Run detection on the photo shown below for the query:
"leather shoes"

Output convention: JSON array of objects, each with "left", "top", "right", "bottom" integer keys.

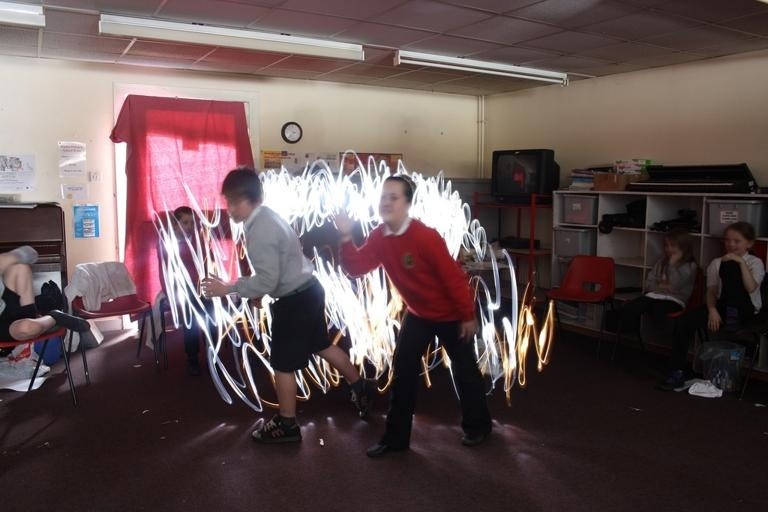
[
  {"left": 461, "top": 434, "right": 484, "bottom": 447},
  {"left": 365, "top": 441, "right": 389, "bottom": 459}
]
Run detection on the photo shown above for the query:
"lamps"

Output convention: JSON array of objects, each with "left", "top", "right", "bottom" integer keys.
[
  {"left": 97, "top": 14, "right": 365, "bottom": 65},
  {"left": 0, "top": 1, "right": 46, "bottom": 30},
  {"left": 393, "top": 50, "right": 570, "bottom": 87}
]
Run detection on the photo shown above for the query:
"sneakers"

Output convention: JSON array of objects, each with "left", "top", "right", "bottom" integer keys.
[
  {"left": 48, "top": 309, "right": 90, "bottom": 334},
  {"left": 352, "top": 377, "right": 376, "bottom": 419},
  {"left": 251, "top": 412, "right": 301, "bottom": 444}
]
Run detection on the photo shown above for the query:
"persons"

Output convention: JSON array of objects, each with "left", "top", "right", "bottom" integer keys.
[
  {"left": 700, "top": 223, "right": 766, "bottom": 352},
  {"left": 202, "top": 167, "right": 381, "bottom": 445},
  {"left": 326, "top": 172, "right": 493, "bottom": 461},
  {"left": 0, "top": 245, "right": 91, "bottom": 341},
  {"left": 607, "top": 230, "right": 700, "bottom": 378},
  {"left": 158, "top": 207, "right": 224, "bottom": 376}
]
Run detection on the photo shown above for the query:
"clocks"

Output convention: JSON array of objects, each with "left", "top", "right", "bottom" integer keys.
[{"left": 281, "top": 122, "right": 303, "bottom": 144}]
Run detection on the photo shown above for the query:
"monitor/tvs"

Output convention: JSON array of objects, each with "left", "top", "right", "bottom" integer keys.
[{"left": 491, "top": 149, "right": 560, "bottom": 204}]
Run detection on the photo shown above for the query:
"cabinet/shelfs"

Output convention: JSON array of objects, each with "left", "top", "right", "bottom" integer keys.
[
  {"left": 472, "top": 192, "right": 552, "bottom": 322},
  {"left": 552, "top": 192, "right": 768, "bottom": 383}
]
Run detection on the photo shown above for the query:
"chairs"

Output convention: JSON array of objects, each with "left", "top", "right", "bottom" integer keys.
[
  {"left": 541, "top": 254, "right": 620, "bottom": 363},
  {"left": 160, "top": 296, "right": 233, "bottom": 369},
  {"left": 692, "top": 333, "right": 760, "bottom": 402},
  {"left": 0, "top": 320, "right": 79, "bottom": 409},
  {"left": 68, "top": 263, "right": 157, "bottom": 387},
  {"left": 632, "top": 273, "right": 705, "bottom": 381}
]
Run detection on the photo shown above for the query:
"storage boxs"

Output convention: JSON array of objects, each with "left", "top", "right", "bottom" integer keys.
[
  {"left": 553, "top": 227, "right": 596, "bottom": 258},
  {"left": 706, "top": 199, "right": 767, "bottom": 237},
  {"left": 561, "top": 194, "right": 598, "bottom": 226},
  {"left": 558, "top": 257, "right": 570, "bottom": 280}
]
